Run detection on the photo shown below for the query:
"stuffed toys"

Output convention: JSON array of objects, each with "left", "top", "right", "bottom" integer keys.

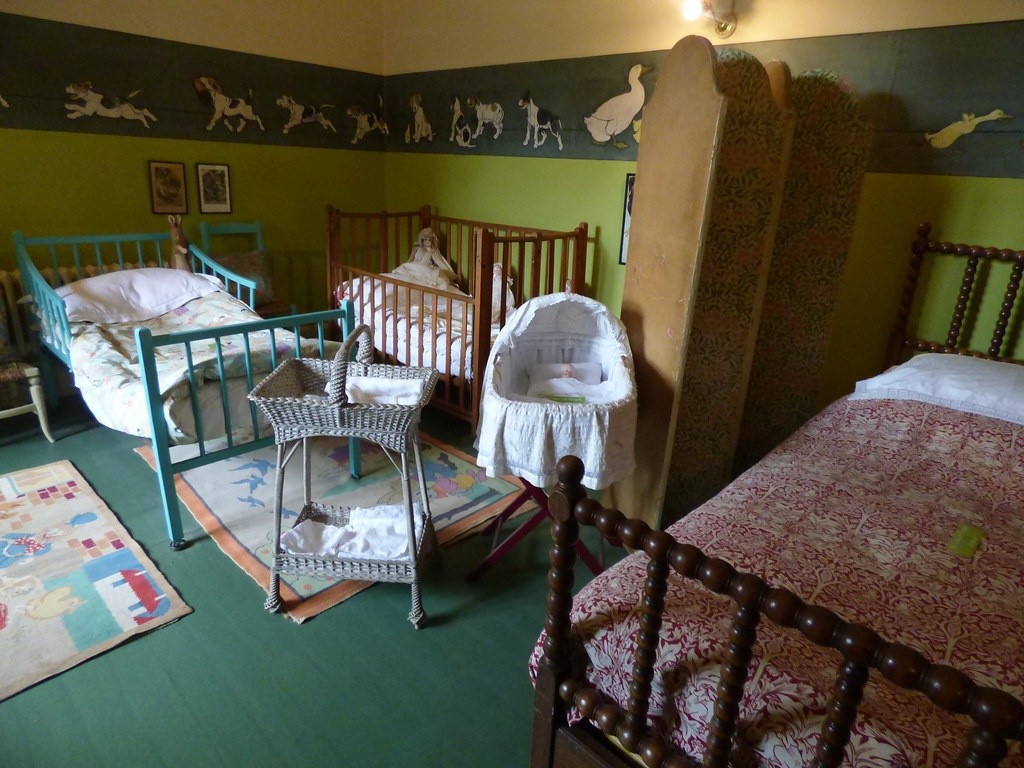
[{"left": 168, "top": 215, "right": 190, "bottom": 272}]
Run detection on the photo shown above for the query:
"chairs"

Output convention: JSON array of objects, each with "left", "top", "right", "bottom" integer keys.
[
  {"left": 0, "top": 301, "right": 55, "bottom": 444},
  {"left": 199, "top": 217, "right": 299, "bottom": 332}
]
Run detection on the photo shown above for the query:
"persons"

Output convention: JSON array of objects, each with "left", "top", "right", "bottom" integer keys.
[
  {"left": 561, "top": 364, "right": 576, "bottom": 378},
  {"left": 565, "top": 280, "right": 572, "bottom": 294},
  {"left": 491, "top": 263, "right": 515, "bottom": 324},
  {"left": 392, "top": 228, "right": 458, "bottom": 290}
]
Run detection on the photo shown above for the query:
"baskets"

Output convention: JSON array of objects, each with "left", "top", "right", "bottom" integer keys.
[
  {"left": 247, "top": 326, "right": 440, "bottom": 454},
  {"left": 275, "top": 503, "right": 430, "bottom": 581}
]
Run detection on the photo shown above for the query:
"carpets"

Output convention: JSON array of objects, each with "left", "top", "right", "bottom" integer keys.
[
  {"left": 133, "top": 433, "right": 550, "bottom": 624},
  {"left": 0, "top": 460, "right": 192, "bottom": 702}
]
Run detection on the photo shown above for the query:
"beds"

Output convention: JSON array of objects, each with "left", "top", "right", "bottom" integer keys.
[
  {"left": 528, "top": 223, "right": 1024, "bottom": 768},
  {"left": 326, "top": 203, "right": 589, "bottom": 440},
  {"left": 337, "top": 273, "right": 472, "bottom": 308},
  {"left": 9, "top": 215, "right": 361, "bottom": 551}
]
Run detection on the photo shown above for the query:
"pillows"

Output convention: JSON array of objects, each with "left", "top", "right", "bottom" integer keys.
[
  {"left": 847, "top": 353, "right": 1024, "bottom": 426},
  {"left": 211, "top": 247, "right": 282, "bottom": 306},
  {"left": 16, "top": 268, "right": 227, "bottom": 325}
]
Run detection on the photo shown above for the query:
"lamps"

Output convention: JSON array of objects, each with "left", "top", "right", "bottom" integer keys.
[{"left": 682, "top": 0, "right": 737, "bottom": 40}]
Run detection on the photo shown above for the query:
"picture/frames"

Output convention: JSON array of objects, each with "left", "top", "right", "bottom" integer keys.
[
  {"left": 148, "top": 160, "right": 188, "bottom": 215},
  {"left": 618, "top": 173, "right": 636, "bottom": 265},
  {"left": 195, "top": 162, "right": 233, "bottom": 215}
]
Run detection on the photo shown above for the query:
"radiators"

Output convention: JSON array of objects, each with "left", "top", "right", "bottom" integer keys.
[{"left": 0, "top": 257, "right": 198, "bottom": 358}]
